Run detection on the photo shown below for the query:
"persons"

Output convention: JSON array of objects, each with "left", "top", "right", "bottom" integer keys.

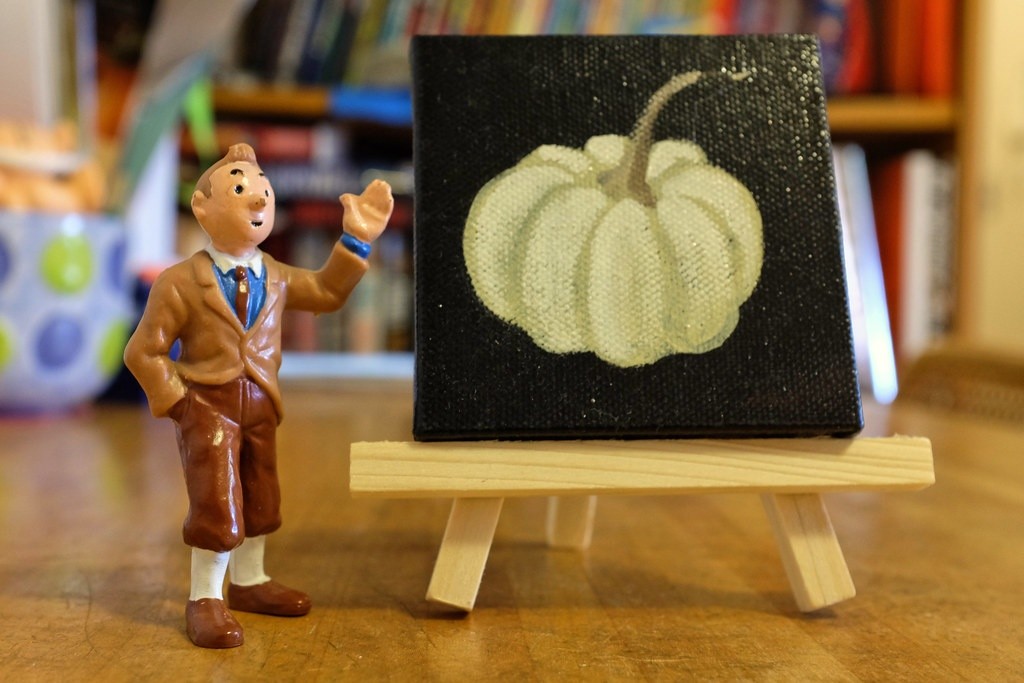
[{"left": 123, "top": 143, "right": 393, "bottom": 648}]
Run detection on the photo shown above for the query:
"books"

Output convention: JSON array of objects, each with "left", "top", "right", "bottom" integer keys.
[{"left": 95, "top": 0, "right": 963, "bottom": 361}]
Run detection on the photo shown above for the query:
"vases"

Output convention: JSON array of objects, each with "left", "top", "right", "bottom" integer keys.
[{"left": 0, "top": 210, "right": 139, "bottom": 417}]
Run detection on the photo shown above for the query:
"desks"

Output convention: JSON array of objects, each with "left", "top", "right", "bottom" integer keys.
[{"left": 0, "top": 375, "right": 1024, "bottom": 683}]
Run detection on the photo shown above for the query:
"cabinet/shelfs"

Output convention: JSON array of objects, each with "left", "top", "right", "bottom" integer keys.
[{"left": 93, "top": 0, "right": 1024, "bottom": 347}]
